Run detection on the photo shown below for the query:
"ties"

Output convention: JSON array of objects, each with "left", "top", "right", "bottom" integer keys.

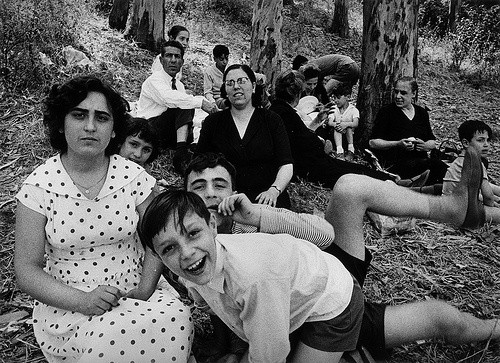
[{"left": 171, "top": 78, "right": 177, "bottom": 90}]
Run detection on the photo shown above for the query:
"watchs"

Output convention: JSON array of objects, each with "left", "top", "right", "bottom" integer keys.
[{"left": 271, "top": 185, "right": 281, "bottom": 195}]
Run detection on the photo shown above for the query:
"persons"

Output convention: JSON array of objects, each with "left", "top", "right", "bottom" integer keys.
[
  {"left": 13, "top": 74, "right": 194, "bottom": 363},
  {"left": 119, "top": 26, "right": 500, "bottom": 363}
]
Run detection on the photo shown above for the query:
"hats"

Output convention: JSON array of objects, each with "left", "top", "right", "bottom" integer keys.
[{"left": 295, "top": 96, "right": 319, "bottom": 114}]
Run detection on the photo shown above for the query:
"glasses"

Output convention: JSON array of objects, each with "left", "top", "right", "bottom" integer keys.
[{"left": 226, "top": 77, "right": 249, "bottom": 87}]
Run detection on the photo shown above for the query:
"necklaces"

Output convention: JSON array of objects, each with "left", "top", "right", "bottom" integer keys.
[{"left": 73, "top": 171, "right": 107, "bottom": 194}]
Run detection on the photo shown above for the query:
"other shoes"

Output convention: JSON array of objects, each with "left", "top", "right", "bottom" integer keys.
[
  {"left": 463, "top": 148, "right": 486, "bottom": 229},
  {"left": 344, "top": 150, "right": 354, "bottom": 161},
  {"left": 336, "top": 153, "right": 346, "bottom": 161}
]
